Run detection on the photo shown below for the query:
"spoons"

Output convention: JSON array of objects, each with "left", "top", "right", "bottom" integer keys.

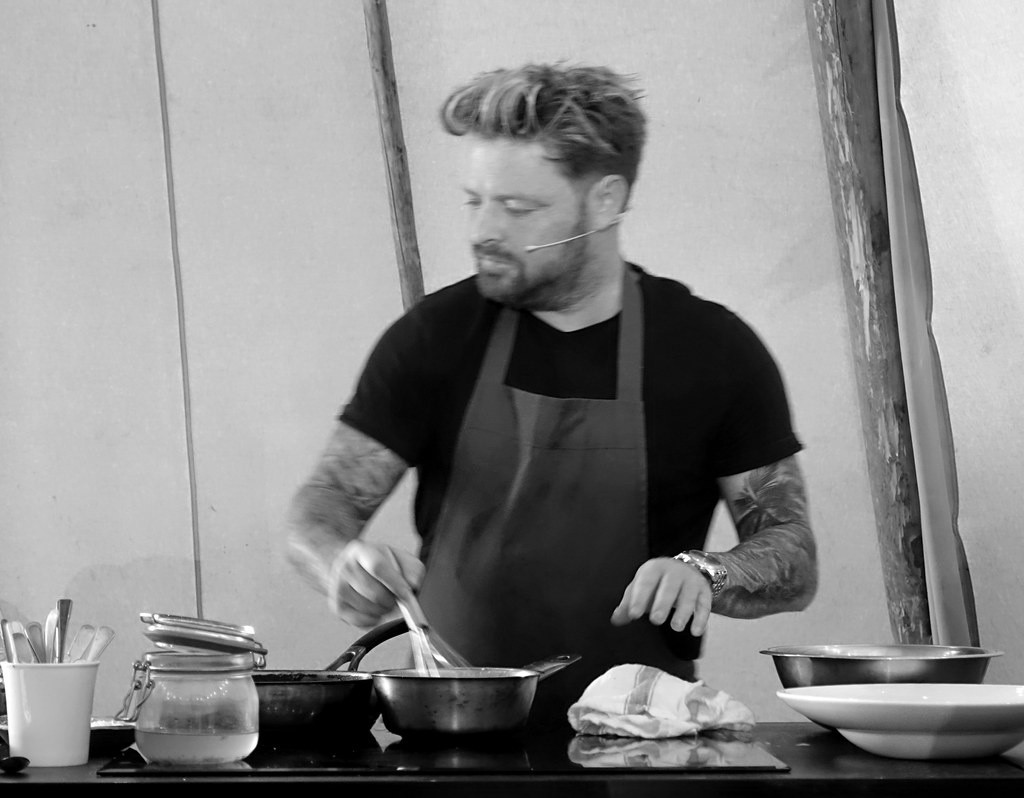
[{"left": 1, "top": 757, "right": 31, "bottom": 773}]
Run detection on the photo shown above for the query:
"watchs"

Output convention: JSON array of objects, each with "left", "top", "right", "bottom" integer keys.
[{"left": 673, "top": 549, "right": 727, "bottom": 599}]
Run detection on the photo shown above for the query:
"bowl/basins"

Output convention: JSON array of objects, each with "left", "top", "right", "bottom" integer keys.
[
  {"left": 759, "top": 642, "right": 1004, "bottom": 689},
  {"left": 776, "top": 682, "right": 1024, "bottom": 760}
]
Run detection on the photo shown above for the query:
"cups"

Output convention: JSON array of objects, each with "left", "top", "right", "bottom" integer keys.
[{"left": 2, "top": 660, "right": 101, "bottom": 768}]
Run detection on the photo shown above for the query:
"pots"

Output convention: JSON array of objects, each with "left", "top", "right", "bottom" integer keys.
[
  {"left": 250, "top": 617, "right": 411, "bottom": 769},
  {"left": 373, "top": 653, "right": 583, "bottom": 739}
]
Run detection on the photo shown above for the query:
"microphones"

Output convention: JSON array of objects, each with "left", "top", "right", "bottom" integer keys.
[{"left": 525, "top": 219, "right": 617, "bottom": 252}]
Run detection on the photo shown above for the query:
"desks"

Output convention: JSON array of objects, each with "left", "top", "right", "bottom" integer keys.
[{"left": 0, "top": 721, "right": 1024, "bottom": 798}]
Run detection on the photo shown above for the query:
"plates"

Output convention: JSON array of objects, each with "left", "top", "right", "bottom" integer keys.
[{"left": 0, "top": 716, "right": 137, "bottom": 758}]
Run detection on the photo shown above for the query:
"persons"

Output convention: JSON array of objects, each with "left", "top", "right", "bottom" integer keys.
[{"left": 283, "top": 66, "right": 818, "bottom": 683}]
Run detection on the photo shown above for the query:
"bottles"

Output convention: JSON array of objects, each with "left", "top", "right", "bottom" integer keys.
[{"left": 112, "top": 613, "right": 267, "bottom": 767}]
